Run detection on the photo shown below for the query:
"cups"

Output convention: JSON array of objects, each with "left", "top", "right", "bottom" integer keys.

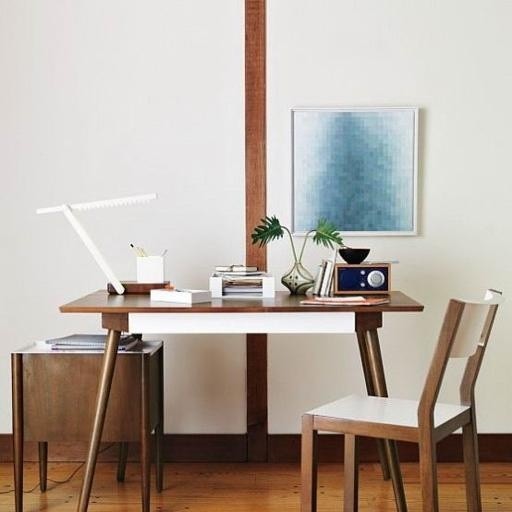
[{"left": 135, "top": 256, "right": 165, "bottom": 285}]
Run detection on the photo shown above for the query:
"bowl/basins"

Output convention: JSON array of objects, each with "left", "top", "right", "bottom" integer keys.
[{"left": 338, "top": 248, "right": 371, "bottom": 266}]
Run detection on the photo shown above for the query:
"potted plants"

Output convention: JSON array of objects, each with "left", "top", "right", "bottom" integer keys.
[{"left": 251, "top": 215, "right": 345, "bottom": 296}]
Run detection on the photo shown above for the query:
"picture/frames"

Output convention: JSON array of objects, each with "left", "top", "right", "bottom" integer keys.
[{"left": 293, "top": 109, "right": 421, "bottom": 238}]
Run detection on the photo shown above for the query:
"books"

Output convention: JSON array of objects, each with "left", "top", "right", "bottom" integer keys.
[
  {"left": 325, "top": 249, "right": 337, "bottom": 295},
  {"left": 45, "top": 337, "right": 140, "bottom": 351},
  {"left": 314, "top": 264, "right": 324, "bottom": 295},
  {"left": 46, "top": 333, "right": 136, "bottom": 347},
  {"left": 318, "top": 258, "right": 332, "bottom": 297},
  {"left": 212, "top": 263, "right": 265, "bottom": 292},
  {"left": 300, "top": 296, "right": 390, "bottom": 306}
]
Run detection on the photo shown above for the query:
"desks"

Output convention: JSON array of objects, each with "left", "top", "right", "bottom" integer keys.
[{"left": 61, "top": 289, "right": 424, "bottom": 507}]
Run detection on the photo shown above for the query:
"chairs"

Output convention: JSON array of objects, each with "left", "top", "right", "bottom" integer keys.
[{"left": 298, "top": 290, "right": 499, "bottom": 512}]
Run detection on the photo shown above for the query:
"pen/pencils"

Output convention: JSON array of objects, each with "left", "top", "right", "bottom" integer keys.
[
  {"left": 160, "top": 248, "right": 167, "bottom": 257},
  {"left": 130, "top": 244, "right": 146, "bottom": 257}
]
